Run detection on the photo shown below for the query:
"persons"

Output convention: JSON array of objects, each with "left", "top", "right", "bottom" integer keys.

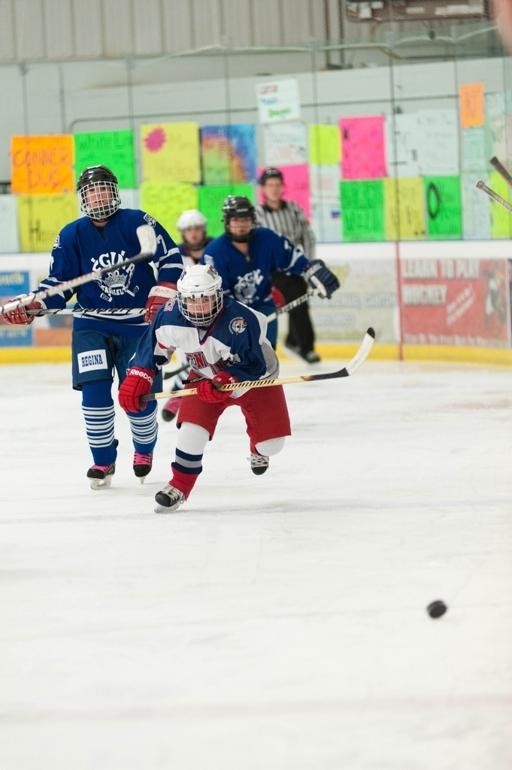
[
  {"left": 170, "top": 207, "right": 216, "bottom": 265},
  {"left": 118, "top": 264, "right": 291, "bottom": 507},
  {"left": 1, "top": 163, "right": 184, "bottom": 479},
  {"left": 161, "top": 194, "right": 340, "bottom": 426},
  {"left": 252, "top": 166, "right": 321, "bottom": 365}
]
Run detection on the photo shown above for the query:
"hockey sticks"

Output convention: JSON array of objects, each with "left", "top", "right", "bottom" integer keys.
[
  {"left": 140, "top": 327, "right": 375, "bottom": 402},
  {"left": 0, "top": 225, "right": 157, "bottom": 314}
]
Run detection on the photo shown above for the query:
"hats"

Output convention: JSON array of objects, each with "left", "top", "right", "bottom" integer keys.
[{"left": 259, "top": 167, "right": 282, "bottom": 185}]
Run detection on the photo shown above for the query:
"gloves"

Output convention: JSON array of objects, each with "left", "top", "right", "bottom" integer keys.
[
  {"left": 144, "top": 281, "right": 176, "bottom": 326},
  {"left": 302, "top": 259, "right": 339, "bottom": 300},
  {"left": 196, "top": 371, "right": 237, "bottom": 404},
  {"left": 0, "top": 292, "right": 47, "bottom": 326},
  {"left": 117, "top": 365, "right": 156, "bottom": 414}
]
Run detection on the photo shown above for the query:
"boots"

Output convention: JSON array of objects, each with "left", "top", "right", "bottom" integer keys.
[
  {"left": 154, "top": 462, "right": 202, "bottom": 507},
  {"left": 249, "top": 453, "right": 269, "bottom": 476},
  {"left": 162, "top": 397, "right": 182, "bottom": 421},
  {"left": 86, "top": 440, "right": 115, "bottom": 479},
  {"left": 284, "top": 336, "right": 320, "bottom": 363},
  {"left": 133, "top": 436, "right": 156, "bottom": 477}
]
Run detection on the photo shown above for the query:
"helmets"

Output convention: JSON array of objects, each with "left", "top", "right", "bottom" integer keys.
[
  {"left": 177, "top": 210, "right": 207, "bottom": 230},
  {"left": 177, "top": 264, "right": 224, "bottom": 328},
  {"left": 75, "top": 164, "right": 121, "bottom": 223},
  {"left": 221, "top": 195, "right": 255, "bottom": 219}
]
